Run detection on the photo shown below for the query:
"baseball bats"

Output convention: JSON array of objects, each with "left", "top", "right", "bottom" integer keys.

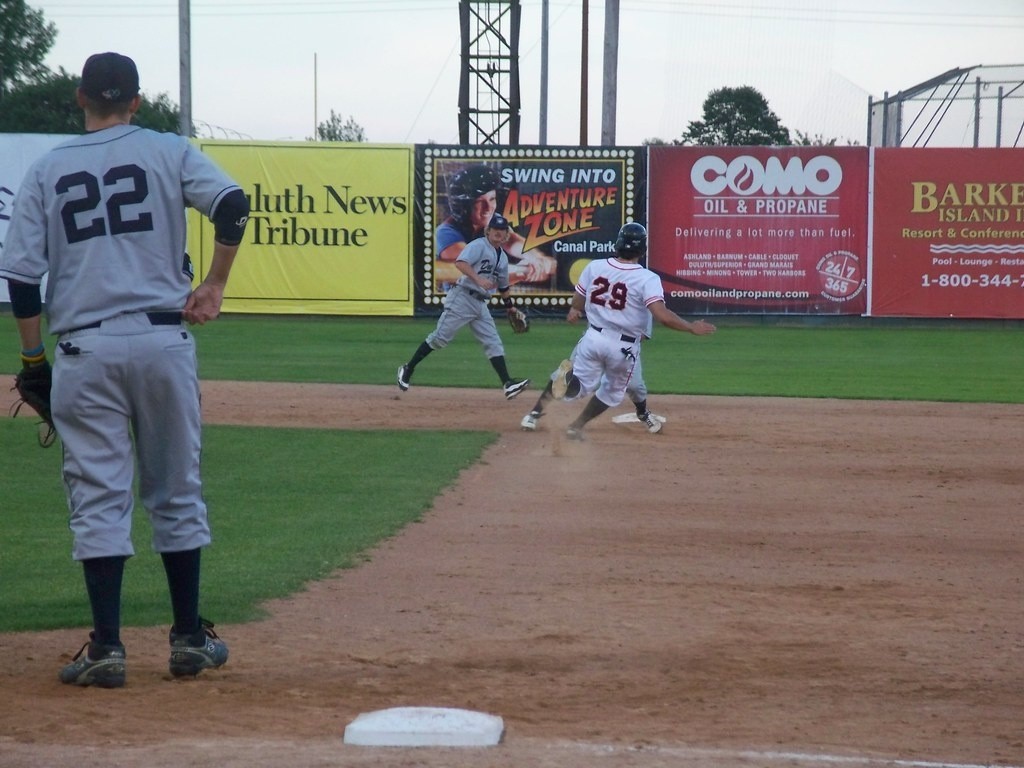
[{"left": 427, "top": 258, "right": 560, "bottom": 285}]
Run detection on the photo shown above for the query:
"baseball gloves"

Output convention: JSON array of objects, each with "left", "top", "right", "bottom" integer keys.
[
  {"left": 508, "top": 309, "right": 530, "bottom": 333},
  {"left": 14, "top": 362, "right": 56, "bottom": 430}
]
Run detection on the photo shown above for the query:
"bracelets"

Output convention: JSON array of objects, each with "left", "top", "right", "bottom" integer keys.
[
  {"left": 23, "top": 342, "right": 44, "bottom": 356},
  {"left": 20, "top": 352, "right": 46, "bottom": 361}
]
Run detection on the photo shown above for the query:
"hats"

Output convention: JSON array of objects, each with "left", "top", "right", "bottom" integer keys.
[
  {"left": 489, "top": 217, "right": 509, "bottom": 231},
  {"left": 79, "top": 52, "right": 140, "bottom": 104}
]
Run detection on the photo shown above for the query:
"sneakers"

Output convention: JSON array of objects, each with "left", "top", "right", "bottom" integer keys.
[
  {"left": 59, "top": 630, "right": 126, "bottom": 689},
  {"left": 637, "top": 409, "right": 662, "bottom": 434},
  {"left": 397, "top": 364, "right": 410, "bottom": 392},
  {"left": 552, "top": 359, "right": 575, "bottom": 399},
  {"left": 520, "top": 410, "right": 539, "bottom": 431},
  {"left": 167, "top": 614, "right": 229, "bottom": 676},
  {"left": 566, "top": 423, "right": 581, "bottom": 438},
  {"left": 503, "top": 376, "right": 530, "bottom": 400}
]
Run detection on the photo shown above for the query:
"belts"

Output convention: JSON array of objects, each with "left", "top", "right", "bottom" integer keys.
[
  {"left": 590, "top": 324, "right": 636, "bottom": 344},
  {"left": 65, "top": 310, "right": 186, "bottom": 331}
]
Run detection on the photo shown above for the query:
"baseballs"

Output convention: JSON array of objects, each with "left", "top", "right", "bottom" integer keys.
[{"left": 569, "top": 257, "right": 594, "bottom": 286}]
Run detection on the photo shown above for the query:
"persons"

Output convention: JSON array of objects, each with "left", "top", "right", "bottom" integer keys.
[
  {"left": 436, "top": 165, "right": 555, "bottom": 293},
  {"left": 550, "top": 223, "right": 717, "bottom": 439},
  {"left": 521, "top": 307, "right": 662, "bottom": 436},
  {"left": 398, "top": 216, "right": 531, "bottom": 401},
  {"left": 0, "top": 52, "right": 249, "bottom": 686}
]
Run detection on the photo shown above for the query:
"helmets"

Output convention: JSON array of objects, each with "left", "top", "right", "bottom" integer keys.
[
  {"left": 448, "top": 165, "right": 503, "bottom": 224},
  {"left": 615, "top": 223, "right": 647, "bottom": 255}
]
